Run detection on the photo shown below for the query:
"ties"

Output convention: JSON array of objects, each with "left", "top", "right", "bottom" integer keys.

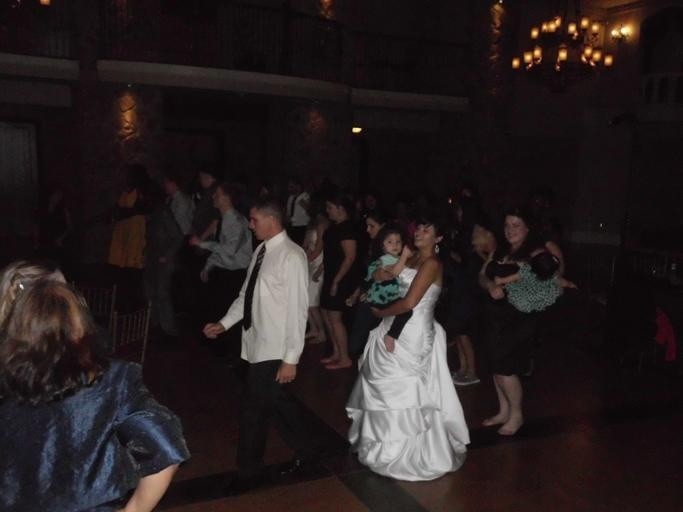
[
  {"left": 243, "top": 244, "right": 266, "bottom": 330},
  {"left": 291, "top": 195, "right": 299, "bottom": 216},
  {"left": 215, "top": 216, "right": 223, "bottom": 244}
]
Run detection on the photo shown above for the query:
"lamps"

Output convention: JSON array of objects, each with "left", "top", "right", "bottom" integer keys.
[
  {"left": 118, "top": 82, "right": 140, "bottom": 143},
  {"left": 611, "top": 24, "right": 631, "bottom": 44},
  {"left": 511, "top": 0, "right": 614, "bottom": 72}
]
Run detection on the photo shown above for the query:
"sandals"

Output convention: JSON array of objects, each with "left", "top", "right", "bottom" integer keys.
[
  {"left": 452, "top": 372, "right": 481, "bottom": 386},
  {"left": 320, "top": 356, "right": 353, "bottom": 369}
]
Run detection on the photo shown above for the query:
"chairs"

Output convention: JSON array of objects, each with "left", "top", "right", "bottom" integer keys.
[
  {"left": 111, "top": 300, "right": 152, "bottom": 365},
  {"left": 70, "top": 281, "right": 117, "bottom": 336}
]
